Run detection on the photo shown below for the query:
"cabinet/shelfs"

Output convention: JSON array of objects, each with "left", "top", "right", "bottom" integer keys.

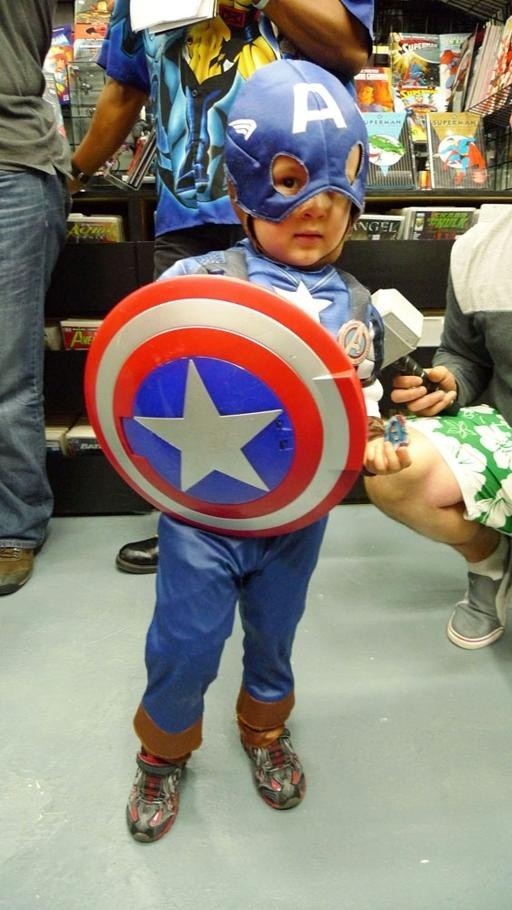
[{"left": 43, "top": 184, "right": 512, "bottom": 517}]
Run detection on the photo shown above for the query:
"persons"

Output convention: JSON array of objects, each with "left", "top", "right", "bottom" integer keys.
[
  {"left": 362, "top": 208, "right": 512, "bottom": 652},
  {"left": 125, "top": 57, "right": 414, "bottom": 843},
  {"left": 1, "top": 0, "right": 73, "bottom": 594},
  {"left": 64, "top": 0, "right": 377, "bottom": 576}
]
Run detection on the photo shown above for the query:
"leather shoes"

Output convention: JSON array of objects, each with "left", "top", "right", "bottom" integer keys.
[{"left": 117, "top": 537, "right": 160, "bottom": 575}]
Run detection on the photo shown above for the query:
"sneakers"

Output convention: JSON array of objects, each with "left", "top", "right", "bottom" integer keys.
[
  {"left": 125, "top": 746, "right": 188, "bottom": 841},
  {"left": 236, "top": 715, "right": 306, "bottom": 808},
  {"left": 0, "top": 536, "right": 51, "bottom": 594},
  {"left": 445, "top": 535, "right": 511, "bottom": 649}
]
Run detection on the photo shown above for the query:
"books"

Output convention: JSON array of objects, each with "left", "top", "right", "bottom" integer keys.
[
  {"left": 145, "top": 0, "right": 219, "bottom": 37},
  {"left": 41, "top": 409, "right": 103, "bottom": 454},
  {"left": 42, "top": 0, "right": 157, "bottom": 190},
  {"left": 345, "top": 13, "right": 512, "bottom": 244},
  {"left": 66, "top": 210, "right": 130, "bottom": 247},
  {"left": 41, "top": 313, "right": 107, "bottom": 353}
]
